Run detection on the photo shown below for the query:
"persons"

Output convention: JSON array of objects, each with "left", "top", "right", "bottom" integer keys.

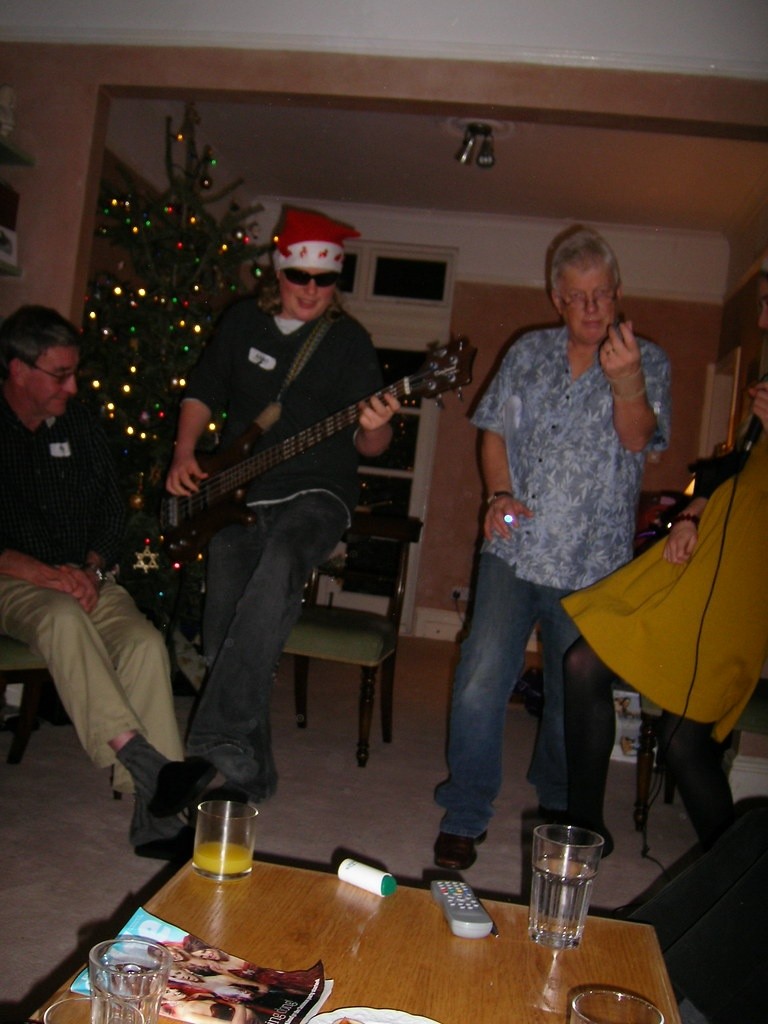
[
  {"left": 1, "top": 304, "right": 244, "bottom": 856},
  {"left": 114, "top": 936, "right": 323, "bottom": 1024},
  {"left": 433, "top": 232, "right": 671, "bottom": 870},
  {"left": 167, "top": 209, "right": 401, "bottom": 801},
  {"left": 561, "top": 259, "right": 768, "bottom": 834}
]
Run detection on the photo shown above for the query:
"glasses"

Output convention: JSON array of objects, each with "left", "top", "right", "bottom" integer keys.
[
  {"left": 22, "top": 359, "right": 77, "bottom": 382},
  {"left": 283, "top": 269, "right": 338, "bottom": 287},
  {"left": 559, "top": 286, "right": 617, "bottom": 310}
]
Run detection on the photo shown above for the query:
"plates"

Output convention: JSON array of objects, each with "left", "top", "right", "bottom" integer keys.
[{"left": 307, "top": 1006, "right": 441, "bottom": 1024}]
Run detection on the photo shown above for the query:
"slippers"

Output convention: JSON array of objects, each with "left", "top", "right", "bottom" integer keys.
[
  {"left": 148, "top": 756, "right": 215, "bottom": 816},
  {"left": 135, "top": 827, "right": 193, "bottom": 860}
]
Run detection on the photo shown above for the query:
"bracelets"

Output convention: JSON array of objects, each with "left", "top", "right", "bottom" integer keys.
[
  {"left": 611, "top": 381, "right": 647, "bottom": 400},
  {"left": 670, "top": 513, "right": 701, "bottom": 529},
  {"left": 487, "top": 491, "right": 513, "bottom": 506}
]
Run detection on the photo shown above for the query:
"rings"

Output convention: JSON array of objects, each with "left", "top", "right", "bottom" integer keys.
[{"left": 606, "top": 349, "right": 615, "bottom": 355}]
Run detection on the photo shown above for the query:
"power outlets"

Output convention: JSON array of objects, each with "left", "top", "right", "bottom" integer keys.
[{"left": 450, "top": 586, "right": 469, "bottom": 601}]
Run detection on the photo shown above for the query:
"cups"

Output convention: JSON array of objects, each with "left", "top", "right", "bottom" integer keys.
[
  {"left": 569, "top": 990, "right": 665, "bottom": 1024},
  {"left": 528, "top": 824, "right": 604, "bottom": 948},
  {"left": 89, "top": 938, "right": 173, "bottom": 1024},
  {"left": 43, "top": 997, "right": 144, "bottom": 1024},
  {"left": 191, "top": 800, "right": 259, "bottom": 881}
]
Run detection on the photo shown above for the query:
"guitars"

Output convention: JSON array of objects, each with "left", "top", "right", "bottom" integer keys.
[{"left": 157, "top": 332, "right": 478, "bottom": 567}]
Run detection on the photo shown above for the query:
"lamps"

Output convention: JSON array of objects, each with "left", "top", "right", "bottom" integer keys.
[{"left": 453, "top": 122, "right": 495, "bottom": 167}]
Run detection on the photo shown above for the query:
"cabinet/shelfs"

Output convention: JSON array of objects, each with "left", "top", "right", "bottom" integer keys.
[{"left": 0, "top": 131, "right": 35, "bottom": 277}]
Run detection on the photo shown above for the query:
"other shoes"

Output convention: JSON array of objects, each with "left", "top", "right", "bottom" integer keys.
[
  {"left": 537, "top": 805, "right": 614, "bottom": 859},
  {"left": 434, "top": 830, "right": 487, "bottom": 869}
]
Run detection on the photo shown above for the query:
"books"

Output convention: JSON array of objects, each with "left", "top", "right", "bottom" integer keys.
[{"left": 70, "top": 906, "right": 334, "bottom": 1024}]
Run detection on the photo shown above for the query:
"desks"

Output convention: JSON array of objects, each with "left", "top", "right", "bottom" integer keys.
[{"left": 21, "top": 857, "right": 683, "bottom": 1024}]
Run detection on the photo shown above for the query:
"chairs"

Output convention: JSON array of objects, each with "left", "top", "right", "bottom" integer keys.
[
  {"left": 0, "top": 635, "right": 55, "bottom": 766},
  {"left": 282, "top": 513, "right": 423, "bottom": 771}
]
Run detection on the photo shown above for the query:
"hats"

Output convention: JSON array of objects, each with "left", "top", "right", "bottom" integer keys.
[{"left": 273, "top": 208, "right": 360, "bottom": 274}]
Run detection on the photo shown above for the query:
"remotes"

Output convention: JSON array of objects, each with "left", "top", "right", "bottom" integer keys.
[{"left": 430, "top": 880, "right": 493, "bottom": 939}]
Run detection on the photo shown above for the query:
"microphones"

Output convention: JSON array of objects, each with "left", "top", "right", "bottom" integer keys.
[{"left": 742, "top": 372, "right": 768, "bottom": 455}]
